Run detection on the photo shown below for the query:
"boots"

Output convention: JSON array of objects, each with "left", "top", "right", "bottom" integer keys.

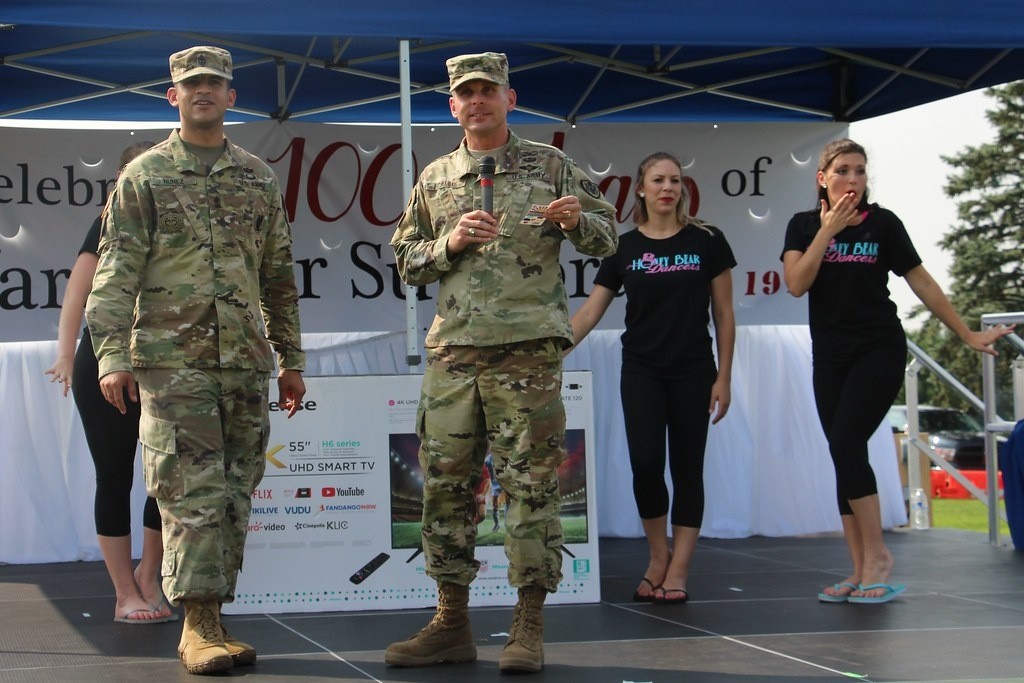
[
  {"left": 220, "top": 624, "right": 257, "bottom": 664},
  {"left": 177, "top": 598, "right": 233, "bottom": 674},
  {"left": 385, "top": 584, "right": 478, "bottom": 666},
  {"left": 498, "top": 586, "right": 544, "bottom": 669}
]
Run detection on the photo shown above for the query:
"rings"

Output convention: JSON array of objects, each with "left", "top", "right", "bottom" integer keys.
[
  {"left": 566, "top": 210, "right": 571, "bottom": 218},
  {"left": 469, "top": 228, "right": 476, "bottom": 237}
]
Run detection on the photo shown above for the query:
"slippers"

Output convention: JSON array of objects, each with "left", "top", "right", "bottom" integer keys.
[
  {"left": 114, "top": 605, "right": 166, "bottom": 624},
  {"left": 847, "top": 583, "right": 904, "bottom": 603},
  {"left": 634, "top": 576, "right": 663, "bottom": 600},
  {"left": 818, "top": 584, "right": 857, "bottom": 601},
  {"left": 149, "top": 597, "right": 179, "bottom": 621},
  {"left": 653, "top": 586, "right": 689, "bottom": 603}
]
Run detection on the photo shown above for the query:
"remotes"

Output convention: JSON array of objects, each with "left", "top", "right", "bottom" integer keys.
[{"left": 350, "top": 553, "right": 391, "bottom": 585}]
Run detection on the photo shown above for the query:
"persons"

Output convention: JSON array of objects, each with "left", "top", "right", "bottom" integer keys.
[
  {"left": 473, "top": 447, "right": 511, "bottom": 532},
  {"left": 781, "top": 139, "right": 1015, "bottom": 604},
  {"left": 85, "top": 46, "right": 306, "bottom": 675},
  {"left": 383, "top": 51, "right": 619, "bottom": 675},
  {"left": 43, "top": 141, "right": 181, "bottom": 622},
  {"left": 563, "top": 151, "right": 738, "bottom": 601}
]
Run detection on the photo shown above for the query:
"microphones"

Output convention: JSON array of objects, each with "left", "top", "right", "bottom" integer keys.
[{"left": 479, "top": 155, "right": 497, "bottom": 225}]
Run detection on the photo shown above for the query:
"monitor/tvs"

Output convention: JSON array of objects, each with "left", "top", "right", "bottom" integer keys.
[{"left": 389, "top": 427, "right": 588, "bottom": 563}]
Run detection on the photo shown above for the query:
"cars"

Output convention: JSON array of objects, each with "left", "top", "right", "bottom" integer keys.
[{"left": 888, "top": 404, "right": 1011, "bottom": 472}]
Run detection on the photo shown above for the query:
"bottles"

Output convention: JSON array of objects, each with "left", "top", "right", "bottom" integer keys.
[{"left": 913, "top": 488, "right": 930, "bottom": 530}]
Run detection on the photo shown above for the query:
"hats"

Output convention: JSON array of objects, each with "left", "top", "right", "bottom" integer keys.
[
  {"left": 446, "top": 52, "right": 510, "bottom": 93},
  {"left": 168, "top": 45, "right": 234, "bottom": 84}
]
